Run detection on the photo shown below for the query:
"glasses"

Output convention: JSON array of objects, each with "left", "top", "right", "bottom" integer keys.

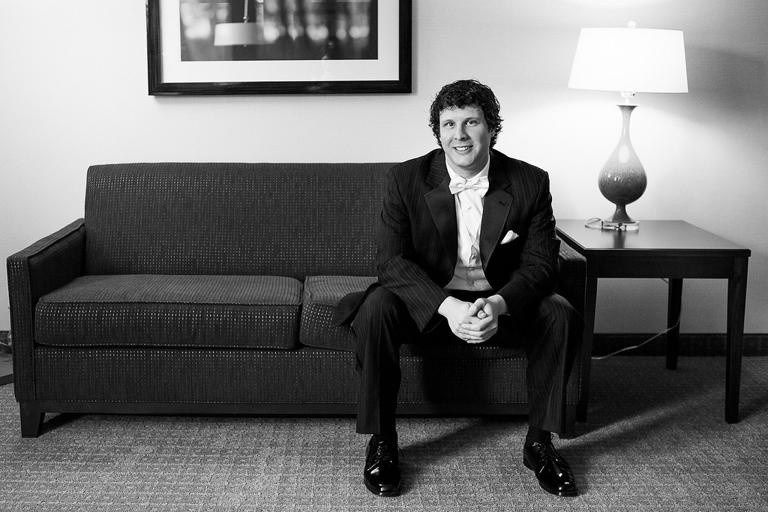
[{"left": 584, "top": 218, "right": 600, "bottom": 230}]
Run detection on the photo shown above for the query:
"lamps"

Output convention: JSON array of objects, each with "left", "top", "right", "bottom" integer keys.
[{"left": 566, "top": 25, "right": 690, "bottom": 231}]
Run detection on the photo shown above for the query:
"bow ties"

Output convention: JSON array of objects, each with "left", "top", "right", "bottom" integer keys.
[{"left": 449, "top": 173, "right": 489, "bottom": 199}]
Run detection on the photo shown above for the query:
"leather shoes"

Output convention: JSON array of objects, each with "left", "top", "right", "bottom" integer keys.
[
  {"left": 523, "top": 438, "right": 578, "bottom": 497},
  {"left": 363, "top": 431, "right": 404, "bottom": 497}
]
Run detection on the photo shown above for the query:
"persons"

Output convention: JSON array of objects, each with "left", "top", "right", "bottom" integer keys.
[{"left": 330, "top": 76, "right": 586, "bottom": 501}]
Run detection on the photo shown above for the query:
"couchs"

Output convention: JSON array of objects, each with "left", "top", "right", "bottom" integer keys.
[{"left": 5, "top": 161, "right": 597, "bottom": 438}]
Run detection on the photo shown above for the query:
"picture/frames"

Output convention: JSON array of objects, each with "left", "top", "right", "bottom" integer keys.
[{"left": 145, "top": 0, "right": 413, "bottom": 94}]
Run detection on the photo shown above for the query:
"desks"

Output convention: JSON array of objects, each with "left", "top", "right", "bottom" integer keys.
[{"left": 553, "top": 220, "right": 753, "bottom": 427}]
